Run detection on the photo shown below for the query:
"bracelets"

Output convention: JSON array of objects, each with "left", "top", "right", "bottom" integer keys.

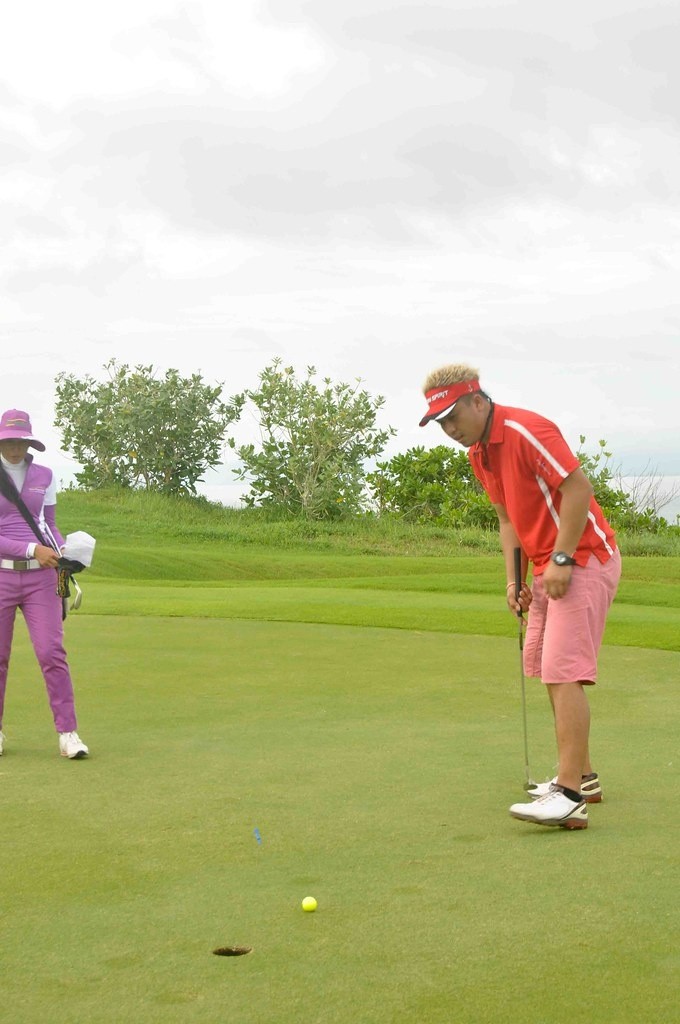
[
  {"left": 506, "top": 582, "right": 525, "bottom": 589},
  {"left": 29, "top": 543, "right": 37, "bottom": 557}
]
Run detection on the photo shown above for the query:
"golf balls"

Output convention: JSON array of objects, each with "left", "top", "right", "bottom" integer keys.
[{"left": 302, "top": 896, "right": 318, "bottom": 913}]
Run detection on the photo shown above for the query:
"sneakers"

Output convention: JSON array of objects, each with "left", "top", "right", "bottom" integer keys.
[
  {"left": 58, "top": 731, "right": 88, "bottom": 759},
  {"left": 0, "top": 731, "right": 7, "bottom": 755},
  {"left": 526, "top": 774, "right": 603, "bottom": 803},
  {"left": 509, "top": 783, "right": 588, "bottom": 829}
]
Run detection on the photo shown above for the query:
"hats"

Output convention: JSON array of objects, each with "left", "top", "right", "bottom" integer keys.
[
  {"left": 0, "top": 409, "right": 45, "bottom": 452},
  {"left": 419, "top": 381, "right": 480, "bottom": 426}
]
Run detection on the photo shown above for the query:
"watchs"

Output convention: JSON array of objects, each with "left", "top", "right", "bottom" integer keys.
[{"left": 551, "top": 551, "right": 576, "bottom": 566}]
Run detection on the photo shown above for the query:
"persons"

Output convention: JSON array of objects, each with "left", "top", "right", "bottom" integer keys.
[
  {"left": 0, "top": 409, "right": 88, "bottom": 760},
  {"left": 420, "top": 366, "right": 622, "bottom": 830}
]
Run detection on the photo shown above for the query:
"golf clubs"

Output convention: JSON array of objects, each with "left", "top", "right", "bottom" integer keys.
[
  {"left": 513, "top": 547, "right": 538, "bottom": 791},
  {"left": 44, "top": 521, "right": 82, "bottom": 609}
]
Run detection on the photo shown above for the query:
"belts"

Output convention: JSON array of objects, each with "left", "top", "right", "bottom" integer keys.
[{"left": 1, "top": 559, "right": 40, "bottom": 570}]
